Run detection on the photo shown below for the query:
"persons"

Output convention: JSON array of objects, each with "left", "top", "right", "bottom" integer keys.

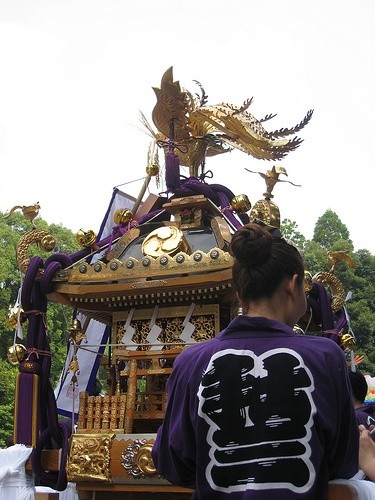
[
  {"left": 329, "top": 370, "right": 375, "bottom": 500},
  {"left": 59, "top": 378, "right": 108, "bottom": 438},
  {"left": 151, "top": 223, "right": 360, "bottom": 500}
]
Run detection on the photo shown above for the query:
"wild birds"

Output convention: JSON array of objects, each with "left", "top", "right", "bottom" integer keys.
[{"left": 151, "top": 66, "right": 314, "bottom": 166}]
